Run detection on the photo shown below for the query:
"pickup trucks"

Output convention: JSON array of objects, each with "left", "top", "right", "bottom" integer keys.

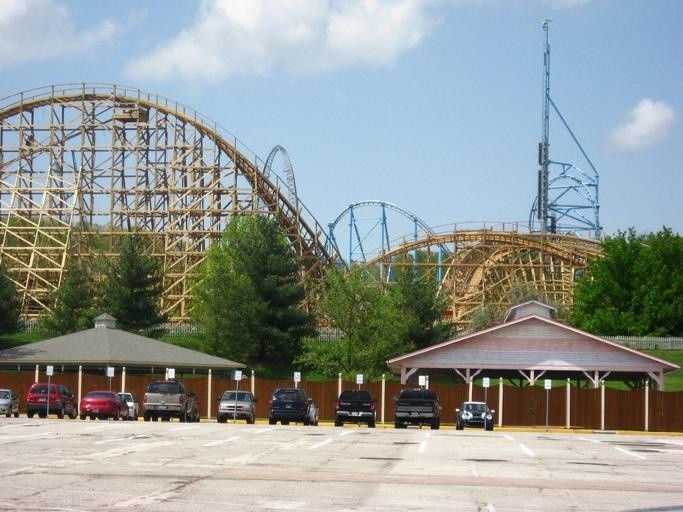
[{"left": 335, "top": 390, "right": 377, "bottom": 428}]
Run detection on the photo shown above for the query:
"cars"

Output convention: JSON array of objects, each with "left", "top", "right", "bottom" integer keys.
[
  {"left": 268, "top": 389, "right": 313, "bottom": 426},
  {"left": 307, "top": 398, "right": 319, "bottom": 424},
  {"left": 0, "top": 381, "right": 199, "bottom": 423},
  {"left": 217, "top": 391, "right": 258, "bottom": 424},
  {"left": 455, "top": 402, "right": 495, "bottom": 430}
]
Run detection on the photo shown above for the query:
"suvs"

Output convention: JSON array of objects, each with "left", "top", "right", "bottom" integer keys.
[{"left": 393, "top": 389, "right": 443, "bottom": 430}]
